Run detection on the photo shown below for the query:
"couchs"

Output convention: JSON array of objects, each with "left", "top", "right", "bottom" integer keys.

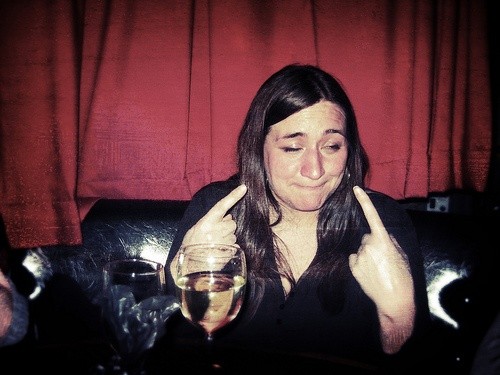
[{"left": 0, "top": 172, "right": 500, "bottom": 375}]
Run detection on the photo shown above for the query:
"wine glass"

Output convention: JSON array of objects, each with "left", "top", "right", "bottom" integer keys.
[
  {"left": 99, "top": 259, "right": 166, "bottom": 375},
  {"left": 176, "top": 244, "right": 247, "bottom": 372}
]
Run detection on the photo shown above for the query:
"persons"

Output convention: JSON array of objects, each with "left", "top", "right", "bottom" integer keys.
[
  {"left": 167, "top": 64, "right": 432, "bottom": 375},
  {"left": 0, "top": 214, "right": 29, "bottom": 375}
]
